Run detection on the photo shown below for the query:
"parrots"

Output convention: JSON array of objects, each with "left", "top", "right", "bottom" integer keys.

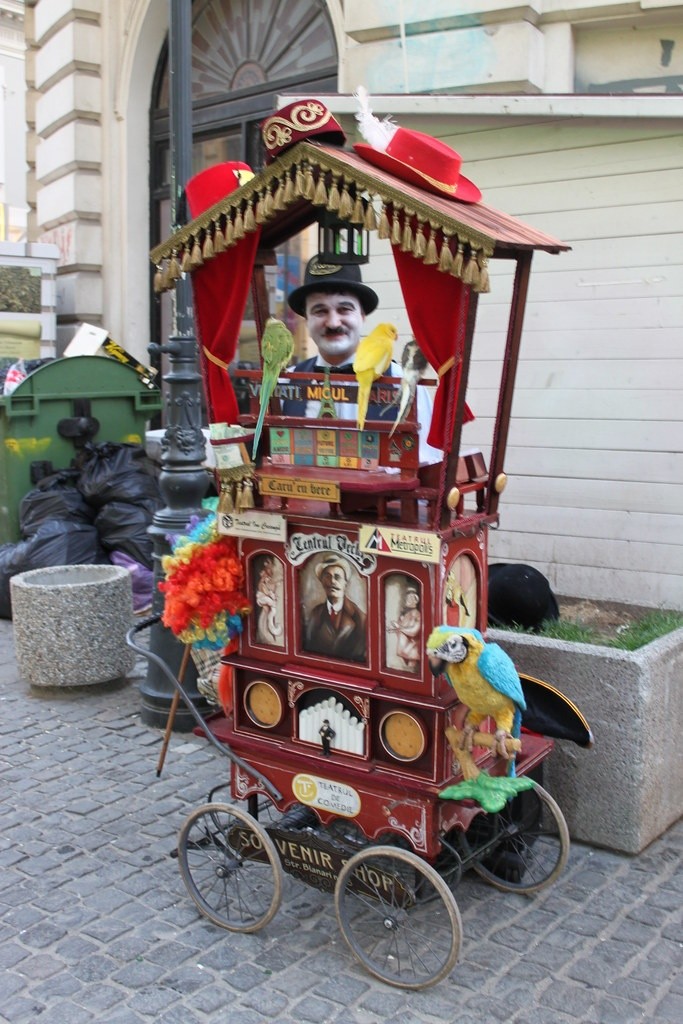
[
  {"left": 425, "top": 624, "right": 527, "bottom": 761},
  {"left": 250, "top": 314, "right": 295, "bottom": 461},
  {"left": 379, "top": 334, "right": 428, "bottom": 440},
  {"left": 351, "top": 322, "right": 398, "bottom": 432}
]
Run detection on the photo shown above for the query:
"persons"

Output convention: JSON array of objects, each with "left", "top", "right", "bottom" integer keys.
[
  {"left": 278, "top": 250, "right": 444, "bottom": 464},
  {"left": 305, "top": 555, "right": 367, "bottom": 659},
  {"left": 319, "top": 719, "right": 335, "bottom": 756}
]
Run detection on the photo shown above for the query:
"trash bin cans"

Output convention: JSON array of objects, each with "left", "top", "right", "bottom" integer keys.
[{"left": 0, "top": 355, "right": 162, "bottom": 544}]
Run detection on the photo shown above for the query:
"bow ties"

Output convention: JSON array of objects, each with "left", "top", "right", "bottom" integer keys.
[{"left": 314, "top": 363, "right": 353, "bottom": 383}]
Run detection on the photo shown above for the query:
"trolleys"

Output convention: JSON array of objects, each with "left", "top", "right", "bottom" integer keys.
[{"left": 124, "top": 83, "right": 596, "bottom": 992}]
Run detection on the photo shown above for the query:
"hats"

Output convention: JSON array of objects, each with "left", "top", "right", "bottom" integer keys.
[
  {"left": 352, "top": 85, "right": 482, "bottom": 204},
  {"left": 260, "top": 99, "right": 346, "bottom": 166},
  {"left": 488, "top": 563, "right": 560, "bottom": 634},
  {"left": 185, "top": 161, "right": 255, "bottom": 219},
  {"left": 519, "top": 673, "right": 594, "bottom": 749},
  {"left": 288, "top": 237, "right": 374, "bottom": 317}
]
